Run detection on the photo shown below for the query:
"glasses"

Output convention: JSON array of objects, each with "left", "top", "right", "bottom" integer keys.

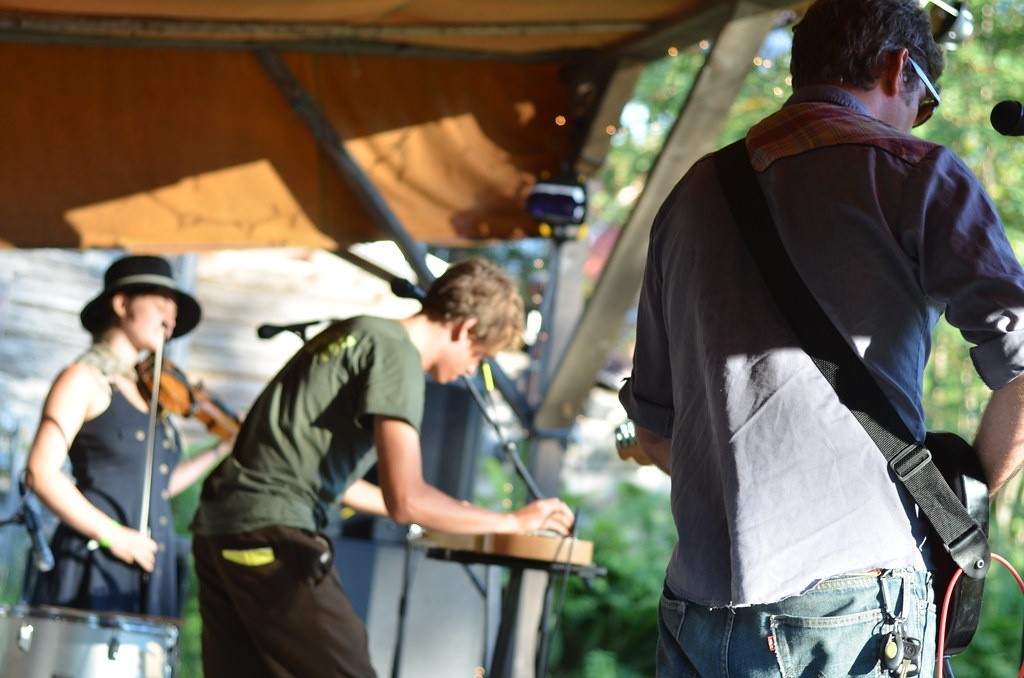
[{"left": 902, "top": 54, "right": 941, "bottom": 130}]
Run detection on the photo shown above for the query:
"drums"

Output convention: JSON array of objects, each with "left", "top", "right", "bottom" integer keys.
[{"left": 0, "top": 601, "right": 184, "bottom": 678}]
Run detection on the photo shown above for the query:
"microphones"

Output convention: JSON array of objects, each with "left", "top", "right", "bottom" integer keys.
[
  {"left": 991, "top": 100, "right": 1024, "bottom": 137},
  {"left": 257, "top": 321, "right": 321, "bottom": 338},
  {"left": 18, "top": 481, "right": 55, "bottom": 571},
  {"left": 391, "top": 278, "right": 426, "bottom": 304}
]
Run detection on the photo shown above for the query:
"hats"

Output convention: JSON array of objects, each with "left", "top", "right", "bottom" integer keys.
[{"left": 79, "top": 255, "right": 202, "bottom": 342}]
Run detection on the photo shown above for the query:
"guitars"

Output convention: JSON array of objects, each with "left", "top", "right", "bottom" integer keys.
[{"left": 611, "top": 426, "right": 991, "bottom": 663}]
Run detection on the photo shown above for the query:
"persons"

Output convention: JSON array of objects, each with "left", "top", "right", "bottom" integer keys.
[
  {"left": 15, "top": 257, "right": 247, "bottom": 678},
  {"left": 191, "top": 257, "right": 577, "bottom": 678},
  {"left": 613, "top": 3, "right": 1024, "bottom": 678}
]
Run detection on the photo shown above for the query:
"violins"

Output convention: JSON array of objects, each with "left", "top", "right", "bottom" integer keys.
[{"left": 139, "top": 352, "right": 245, "bottom": 443}]
